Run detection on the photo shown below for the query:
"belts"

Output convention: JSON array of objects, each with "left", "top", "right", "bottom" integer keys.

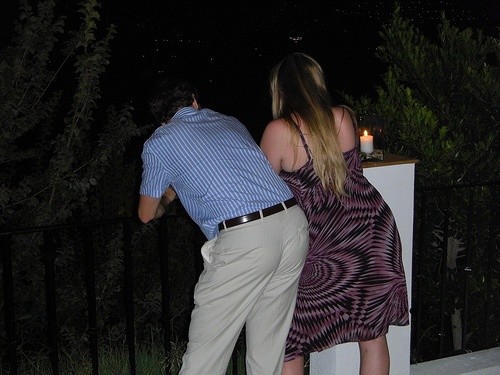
[{"left": 218, "top": 197, "right": 297, "bottom": 233}]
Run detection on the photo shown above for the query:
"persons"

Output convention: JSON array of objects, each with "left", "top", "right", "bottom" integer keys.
[
  {"left": 261, "top": 53, "right": 409, "bottom": 375},
  {"left": 137, "top": 76, "right": 309, "bottom": 375}
]
